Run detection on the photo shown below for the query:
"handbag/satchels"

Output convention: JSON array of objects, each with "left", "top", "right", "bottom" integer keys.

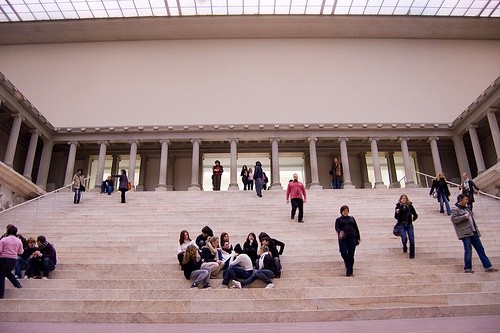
[
  {"left": 80, "top": 185, "right": 85, "bottom": 191},
  {"left": 128, "top": 183, "right": 131, "bottom": 190},
  {"left": 265, "top": 177, "right": 268, "bottom": 183},
  {"left": 329, "top": 171, "right": 333, "bottom": 175},
  {"left": 433, "top": 191, "right": 437, "bottom": 199},
  {"left": 393, "top": 223, "right": 400, "bottom": 236}
]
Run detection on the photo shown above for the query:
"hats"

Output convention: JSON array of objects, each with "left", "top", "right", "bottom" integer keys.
[{"left": 234, "top": 244, "right": 241, "bottom": 251}]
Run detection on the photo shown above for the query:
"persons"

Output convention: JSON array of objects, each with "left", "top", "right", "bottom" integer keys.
[
  {"left": 177, "top": 226, "right": 285, "bottom": 289},
  {"left": 110, "top": 169, "right": 128, "bottom": 203},
  {"left": 458, "top": 173, "right": 482, "bottom": 213},
  {"left": 335, "top": 205, "right": 361, "bottom": 276},
  {"left": 212, "top": 160, "right": 223, "bottom": 190},
  {"left": 430, "top": 172, "right": 451, "bottom": 215},
  {"left": 241, "top": 161, "right": 268, "bottom": 197},
  {"left": 23, "top": 236, "right": 57, "bottom": 279},
  {"left": 72, "top": 169, "right": 85, "bottom": 204},
  {"left": 286, "top": 174, "right": 306, "bottom": 222},
  {"left": 331, "top": 157, "right": 343, "bottom": 189},
  {"left": 451, "top": 194, "right": 498, "bottom": 274},
  {"left": 394, "top": 194, "right": 418, "bottom": 258},
  {"left": 100, "top": 176, "right": 114, "bottom": 195},
  {"left": 0, "top": 226, "right": 24, "bottom": 299},
  {"left": 0, "top": 224, "right": 43, "bottom": 279}
]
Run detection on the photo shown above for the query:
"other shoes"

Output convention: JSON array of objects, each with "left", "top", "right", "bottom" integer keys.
[
  {"left": 465, "top": 271, "right": 474, "bottom": 273},
  {"left": 232, "top": 280, "right": 241, "bottom": 289},
  {"left": 485, "top": 268, "right": 498, "bottom": 272},
  {"left": 18, "top": 285, "right": 23, "bottom": 288},
  {"left": 265, "top": 283, "right": 275, "bottom": 288},
  {"left": 191, "top": 284, "right": 199, "bottom": 289},
  {"left": 34, "top": 275, "right": 42, "bottom": 279},
  {"left": 410, "top": 256, "right": 415, "bottom": 259},
  {"left": 403, "top": 248, "right": 407, "bottom": 253},
  {"left": 42, "top": 277, "right": 47, "bottom": 280},
  {"left": 204, "top": 285, "right": 212, "bottom": 289}
]
